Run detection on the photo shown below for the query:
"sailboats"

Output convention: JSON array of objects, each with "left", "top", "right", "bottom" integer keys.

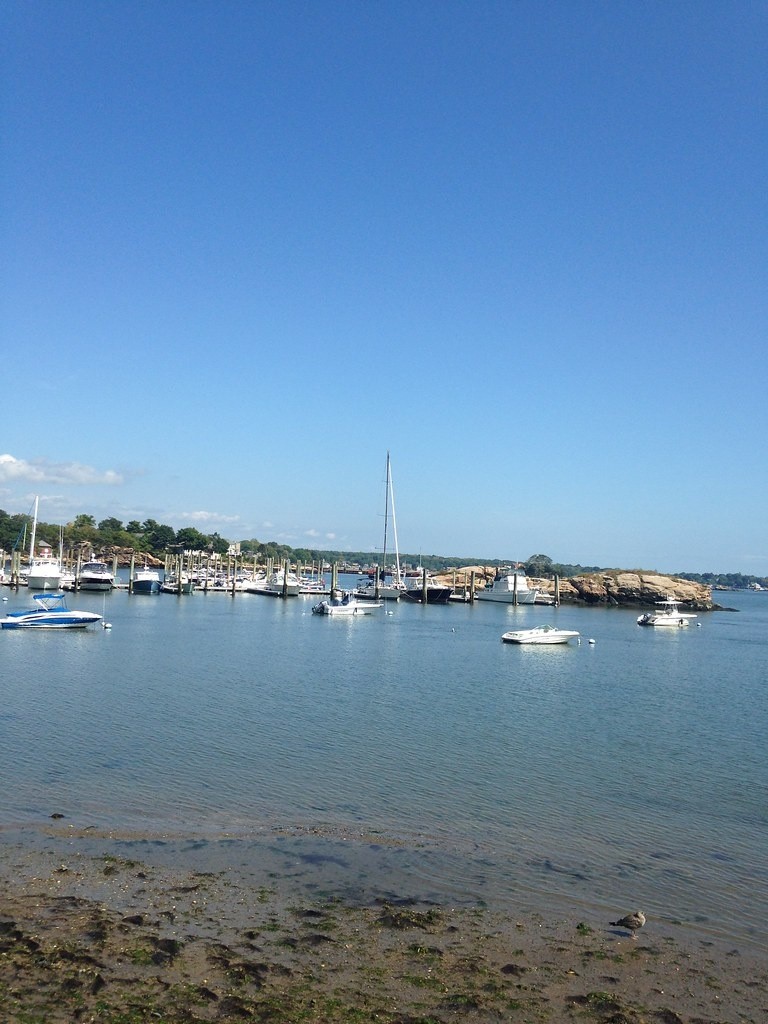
[
  {"left": 25, "top": 495, "right": 62, "bottom": 589},
  {"left": 354, "top": 448, "right": 454, "bottom": 603}
]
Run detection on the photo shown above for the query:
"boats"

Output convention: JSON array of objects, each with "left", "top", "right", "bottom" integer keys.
[
  {"left": 132, "top": 553, "right": 163, "bottom": 596},
  {"left": 180, "top": 583, "right": 195, "bottom": 594},
  {"left": 0, "top": 594, "right": 104, "bottom": 632},
  {"left": 531, "top": 588, "right": 556, "bottom": 607},
  {"left": 477, "top": 560, "right": 538, "bottom": 606},
  {"left": 500, "top": 624, "right": 582, "bottom": 645},
  {"left": 636, "top": 592, "right": 698, "bottom": 626},
  {"left": 79, "top": 552, "right": 115, "bottom": 592},
  {"left": 267, "top": 571, "right": 304, "bottom": 598},
  {"left": 311, "top": 589, "right": 384, "bottom": 617}
]
